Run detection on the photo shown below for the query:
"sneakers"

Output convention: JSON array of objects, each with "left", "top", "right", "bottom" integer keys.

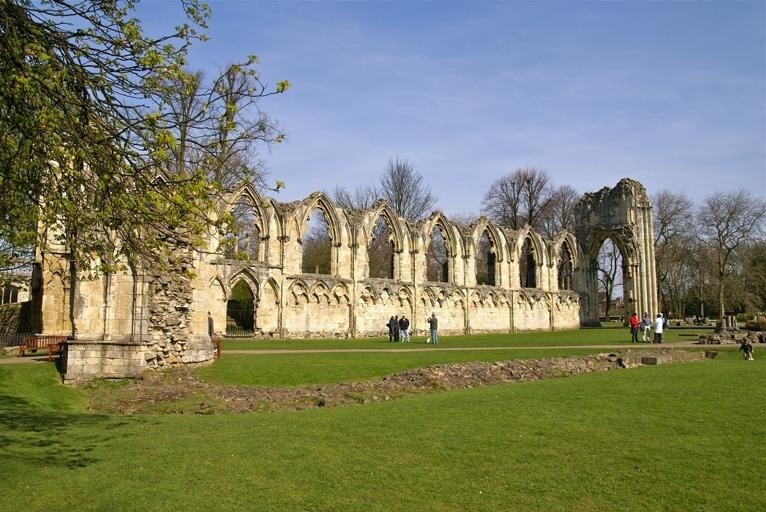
[{"left": 749, "top": 358, "right": 754, "bottom": 360}]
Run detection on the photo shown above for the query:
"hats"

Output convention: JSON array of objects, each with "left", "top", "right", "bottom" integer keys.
[
  {"left": 402, "top": 314, "right": 405, "bottom": 317},
  {"left": 645, "top": 312, "right": 648, "bottom": 315}
]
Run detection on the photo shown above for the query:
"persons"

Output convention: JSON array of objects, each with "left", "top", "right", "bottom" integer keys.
[
  {"left": 631, "top": 311, "right": 638, "bottom": 342},
  {"left": 642, "top": 313, "right": 652, "bottom": 344},
  {"left": 654, "top": 312, "right": 665, "bottom": 344},
  {"left": 427, "top": 313, "right": 439, "bottom": 344},
  {"left": 386, "top": 314, "right": 409, "bottom": 343},
  {"left": 739, "top": 339, "right": 755, "bottom": 361}
]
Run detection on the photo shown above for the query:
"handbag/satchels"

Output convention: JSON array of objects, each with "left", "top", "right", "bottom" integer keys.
[
  {"left": 630, "top": 328, "right": 634, "bottom": 333},
  {"left": 386, "top": 323, "right": 390, "bottom": 326},
  {"left": 640, "top": 324, "right": 645, "bottom": 331}
]
